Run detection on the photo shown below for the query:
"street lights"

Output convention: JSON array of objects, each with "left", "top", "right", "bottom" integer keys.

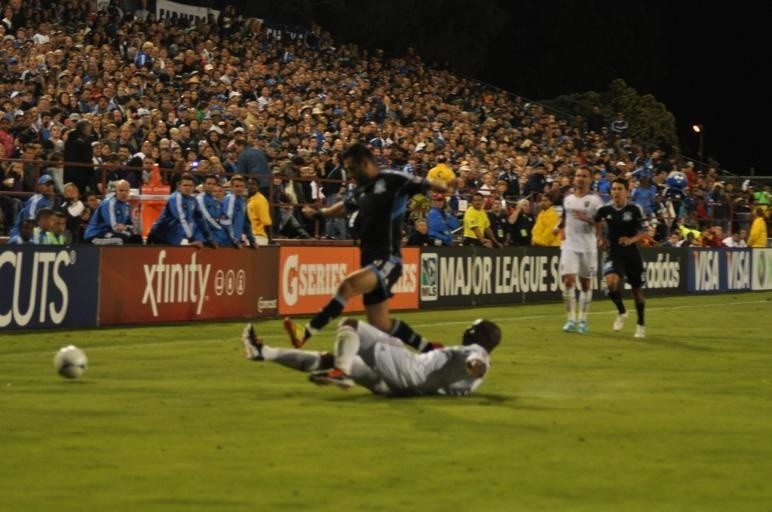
[{"left": 690, "top": 123, "right": 707, "bottom": 167}]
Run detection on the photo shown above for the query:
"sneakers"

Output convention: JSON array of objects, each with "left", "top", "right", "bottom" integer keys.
[
  {"left": 284, "top": 316, "right": 308, "bottom": 348},
  {"left": 577, "top": 319, "right": 588, "bottom": 333},
  {"left": 634, "top": 324, "right": 646, "bottom": 338},
  {"left": 241, "top": 323, "right": 264, "bottom": 361},
  {"left": 563, "top": 320, "right": 576, "bottom": 332},
  {"left": 309, "top": 367, "right": 355, "bottom": 391},
  {"left": 613, "top": 309, "right": 629, "bottom": 331},
  {"left": 431, "top": 342, "right": 443, "bottom": 349}
]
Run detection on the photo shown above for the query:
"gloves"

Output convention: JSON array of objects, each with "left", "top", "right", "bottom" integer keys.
[
  {"left": 208, "top": 239, "right": 219, "bottom": 249},
  {"left": 250, "top": 239, "right": 257, "bottom": 248},
  {"left": 232, "top": 238, "right": 243, "bottom": 249}
]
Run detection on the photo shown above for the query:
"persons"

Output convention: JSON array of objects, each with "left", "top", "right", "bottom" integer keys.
[
  {"left": 1, "top": 1, "right": 771, "bottom": 249},
  {"left": 593, "top": 178, "right": 649, "bottom": 338},
  {"left": 242, "top": 316, "right": 502, "bottom": 396},
  {"left": 284, "top": 142, "right": 466, "bottom": 354},
  {"left": 552, "top": 166, "right": 606, "bottom": 336}
]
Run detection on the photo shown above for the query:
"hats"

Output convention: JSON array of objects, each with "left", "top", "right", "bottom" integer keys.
[
  {"left": 58, "top": 44, "right": 66, "bottom": 49},
  {"left": 600, "top": 151, "right": 608, "bottom": 157},
  {"left": 9, "top": 90, "right": 22, "bottom": 99},
  {"left": 38, "top": 174, "right": 55, "bottom": 184},
  {"left": 90, "top": 90, "right": 102, "bottom": 98},
  {"left": 210, "top": 110, "right": 221, "bottom": 118},
  {"left": 459, "top": 166, "right": 471, "bottom": 172},
  {"left": 177, "top": 105, "right": 187, "bottom": 112},
  {"left": 69, "top": 113, "right": 81, "bottom": 120},
  {"left": 232, "top": 127, "right": 245, "bottom": 134},
  {"left": 58, "top": 72, "right": 67, "bottom": 79},
  {"left": 23, "top": 40, "right": 34, "bottom": 45},
  {"left": 300, "top": 105, "right": 312, "bottom": 114},
  {"left": 748, "top": 183, "right": 756, "bottom": 190},
  {"left": 224, "top": 112, "right": 235, "bottom": 121},
  {"left": 230, "top": 91, "right": 242, "bottom": 98},
  {"left": 116, "top": 30, "right": 126, "bottom": 36},
  {"left": 205, "top": 64, "right": 214, "bottom": 71},
  {"left": 487, "top": 117, "right": 496, "bottom": 123},
  {"left": 8, "top": 57, "right": 18, "bottom": 64},
  {"left": 616, "top": 162, "right": 626, "bottom": 167},
  {"left": 185, "top": 76, "right": 202, "bottom": 85},
  {"left": 142, "top": 42, "right": 153, "bottom": 51},
  {"left": 210, "top": 106, "right": 224, "bottom": 110},
  {"left": 312, "top": 108, "right": 325, "bottom": 115},
  {"left": 687, "top": 162, "right": 695, "bottom": 168},
  {"left": 413, "top": 147, "right": 424, "bottom": 153}
]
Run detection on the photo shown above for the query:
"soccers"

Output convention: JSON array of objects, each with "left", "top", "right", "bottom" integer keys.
[{"left": 54, "top": 345, "right": 86, "bottom": 379}]
